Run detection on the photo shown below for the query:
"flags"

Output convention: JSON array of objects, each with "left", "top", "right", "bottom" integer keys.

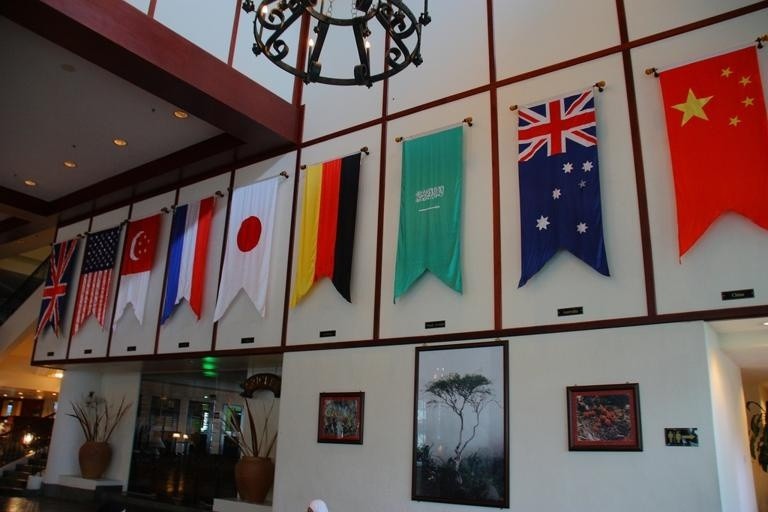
[
  {"left": 213, "top": 176, "right": 279, "bottom": 321},
  {"left": 113, "top": 214, "right": 161, "bottom": 331},
  {"left": 656, "top": 42, "right": 768, "bottom": 264},
  {"left": 34, "top": 238, "right": 79, "bottom": 337},
  {"left": 72, "top": 226, "right": 121, "bottom": 337},
  {"left": 160, "top": 196, "right": 217, "bottom": 323},
  {"left": 288, "top": 152, "right": 361, "bottom": 308},
  {"left": 516, "top": 86, "right": 611, "bottom": 290},
  {"left": 393, "top": 125, "right": 464, "bottom": 305}
]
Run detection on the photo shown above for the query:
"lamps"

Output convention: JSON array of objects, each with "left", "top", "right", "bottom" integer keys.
[{"left": 241, "top": 0, "right": 433, "bottom": 90}]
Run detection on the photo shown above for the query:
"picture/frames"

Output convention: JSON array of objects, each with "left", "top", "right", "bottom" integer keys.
[
  {"left": 410, "top": 339, "right": 511, "bottom": 508},
  {"left": 565, "top": 383, "right": 643, "bottom": 453},
  {"left": 316, "top": 391, "right": 365, "bottom": 446}
]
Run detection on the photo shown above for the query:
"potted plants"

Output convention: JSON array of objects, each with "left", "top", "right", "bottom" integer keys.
[
  {"left": 63, "top": 394, "right": 134, "bottom": 479},
  {"left": 214, "top": 396, "right": 277, "bottom": 505}
]
